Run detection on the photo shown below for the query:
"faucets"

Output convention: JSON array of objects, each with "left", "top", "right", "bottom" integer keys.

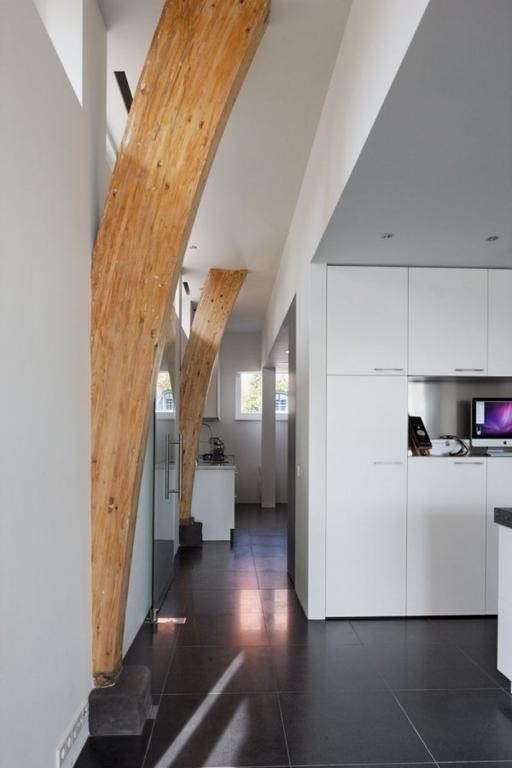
[{"left": 202, "top": 423, "right": 213, "bottom": 437}]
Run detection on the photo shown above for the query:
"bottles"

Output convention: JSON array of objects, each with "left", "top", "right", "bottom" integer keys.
[{"left": 213, "top": 438, "right": 224, "bottom": 459}]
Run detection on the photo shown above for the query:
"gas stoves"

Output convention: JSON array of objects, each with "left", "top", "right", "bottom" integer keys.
[{"left": 198, "top": 454, "right": 230, "bottom": 464}]
